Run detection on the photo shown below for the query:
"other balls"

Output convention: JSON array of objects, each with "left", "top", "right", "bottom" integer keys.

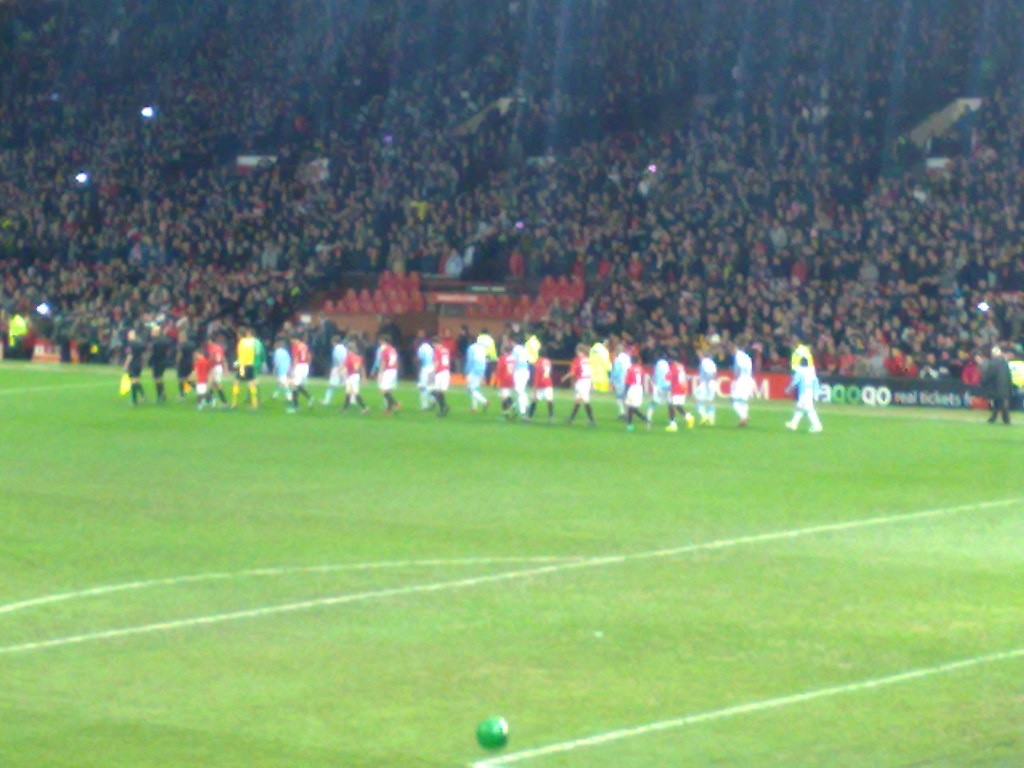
[{"left": 476, "top": 714, "right": 511, "bottom": 749}]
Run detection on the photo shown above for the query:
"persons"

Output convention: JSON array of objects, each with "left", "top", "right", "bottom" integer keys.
[
  {"left": 462, "top": 336, "right": 492, "bottom": 414},
  {"left": 412, "top": 339, "right": 436, "bottom": 412},
  {"left": 145, "top": 326, "right": 171, "bottom": 406},
  {"left": 509, "top": 333, "right": 532, "bottom": 415},
  {"left": 479, "top": 324, "right": 498, "bottom": 384},
  {"left": 320, "top": 335, "right": 348, "bottom": 405},
  {"left": 175, "top": 330, "right": 197, "bottom": 401},
  {"left": 287, "top": 335, "right": 315, "bottom": 414},
  {"left": 188, "top": 349, "right": 216, "bottom": 413},
  {"left": 229, "top": 328, "right": 268, "bottom": 411},
  {"left": 121, "top": 329, "right": 148, "bottom": 406},
  {"left": 430, "top": 337, "right": 451, "bottom": 417},
  {"left": 338, "top": 340, "right": 370, "bottom": 416},
  {"left": 205, "top": 337, "right": 231, "bottom": 407},
  {"left": 980, "top": 345, "right": 1013, "bottom": 424},
  {"left": 368, "top": 334, "right": 401, "bottom": 414},
  {"left": 272, "top": 343, "right": 296, "bottom": 414},
  {"left": 526, "top": 329, "right": 822, "bottom": 435},
  {"left": 1, "top": 0, "right": 1024, "bottom": 383},
  {"left": 492, "top": 342, "right": 519, "bottom": 419}
]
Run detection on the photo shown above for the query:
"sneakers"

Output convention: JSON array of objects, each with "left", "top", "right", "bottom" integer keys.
[
  {"left": 784, "top": 421, "right": 798, "bottom": 431},
  {"left": 807, "top": 423, "right": 823, "bottom": 434}
]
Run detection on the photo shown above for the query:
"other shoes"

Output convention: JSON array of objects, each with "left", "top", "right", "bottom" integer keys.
[
  {"left": 502, "top": 409, "right": 519, "bottom": 421},
  {"left": 646, "top": 419, "right": 654, "bottom": 430},
  {"left": 286, "top": 407, "right": 298, "bottom": 415},
  {"left": 360, "top": 406, "right": 370, "bottom": 414},
  {"left": 545, "top": 417, "right": 555, "bottom": 426},
  {"left": 339, "top": 405, "right": 351, "bottom": 414},
  {"left": 738, "top": 418, "right": 748, "bottom": 428},
  {"left": 219, "top": 403, "right": 237, "bottom": 412},
  {"left": 437, "top": 406, "right": 450, "bottom": 418},
  {"left": 520, "top": 415, "right": 531, "bottom": 424},
  {"left": 587, "top": 421, "right": 596, "bottom": 427},
  {"left": 483, "top": 402, "right": 490, "bottom": 412},
  {"left": 306, "top": 397, "right": 317, "bottom": 411},
  {"left": 430, "top": 400, "right": 436, "bottom": 410},
  {"left": 140, "top": 396, "right": 148, "bottom": 406},
  {"left": 665, "top": 423, "right": 678, "bottom": 433},
  {"left": 625, "top": 424, "right": 635, "bottom": 431},
  {"left": 685, "top": 415, "right": 695, "bottom": 430},
  {"left": 385, "top": 402, "right": 401, "bottom": 416},
  {"left": 420, "top": 402, "right": 428, "bottom": 412},
  {"left": 267, "top": 393, "right": 279, "bottom": 401},
  {"left": 464, "top": 410, "right": 477, "bottom": 415},
  {"left": 128, "top": 398, "right": 138, "bottom": 409},
  {"left": 319, "top": 399, "right": 329, "bottom": 407},
  {"left": 197, "top": 404, "right": 203, "bottom": 412},
  {"left": 699, "top": 415, "right": 716, "bottom": 426},
  {"left": 156, "top": 393, "right": 168, "bottom": 405},
  {"left": 211, "top": 400, "right": 217, "bottom": 408},
  {"left": 246, "top": 405, "right": 258, "bottom": 413},
  {"left": 564, "top": 417, "right": 575, "bottom": 425}
]
